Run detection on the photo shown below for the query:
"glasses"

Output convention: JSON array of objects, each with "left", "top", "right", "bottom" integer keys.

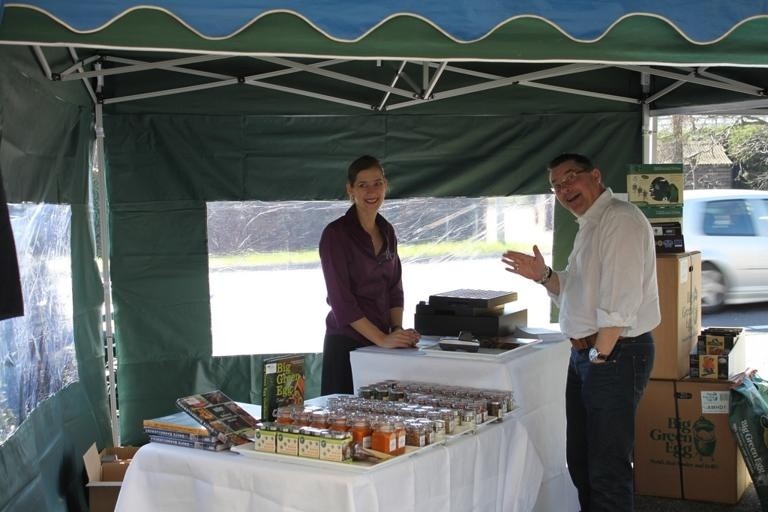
[{"left": 550, "top": 168, "right": 587, "bottom": 194}]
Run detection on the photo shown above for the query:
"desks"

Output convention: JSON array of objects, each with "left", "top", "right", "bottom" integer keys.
[
  {"left": 353, "top": 328, "right": 582, "bottom": 509},
  {"left": 141, "top": 396, "right": 519, "bottom": 512}
]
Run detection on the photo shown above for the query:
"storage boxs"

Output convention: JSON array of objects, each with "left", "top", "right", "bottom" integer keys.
[
  {"left": 82, "top": 441, "right": 131, "bottom": 512},
  {"left": 631, "top": 380, "right": 754, "bottom": 504},
  {"left": 650, "top": 248, "right": 703, "bottom": 379}
]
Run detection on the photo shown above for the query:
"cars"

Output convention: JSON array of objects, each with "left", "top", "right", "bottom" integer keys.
[{"left": 682, "top": 188, "right": 768, "bottom": 312}]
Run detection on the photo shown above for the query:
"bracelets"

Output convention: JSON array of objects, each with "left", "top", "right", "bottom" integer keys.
[
  {"left": 535, "top": 266, "right": 553, "bottom": 286},
  {"left": 388, "top": 325, "right": 405, "bottom": 334}
]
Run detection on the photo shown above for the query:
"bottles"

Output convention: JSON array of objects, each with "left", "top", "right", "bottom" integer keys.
[
  {"left": 272, "top": 404, "right": 312, "bottom": 427},
  {"left": 325, "top": 377, "right": 515, "bottom": 456},
  {"left": 309, "top": 411, "right": 328, "bottom": 430}
]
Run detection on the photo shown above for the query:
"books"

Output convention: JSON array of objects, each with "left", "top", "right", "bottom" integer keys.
[
  {"left": 261, "top": 354, "right": 305, "bottom": 423},
  {"left": 176, "top": 390, "right": 267, "bottom": 450},
  {"left": 143, "top": 411, "right": 230, "bottom": 452}
]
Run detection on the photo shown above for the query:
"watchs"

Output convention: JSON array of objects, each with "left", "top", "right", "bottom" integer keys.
[{"left": 588, "top": 346, "right": 610, "bottom": 365}]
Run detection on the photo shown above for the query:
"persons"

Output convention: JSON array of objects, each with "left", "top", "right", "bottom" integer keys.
[
  {"left": 501, "top": 154, "right": 661, "bottom": 511},
  {"left": 318, "top": 154, "right": 422, "bottom": 395}
]
